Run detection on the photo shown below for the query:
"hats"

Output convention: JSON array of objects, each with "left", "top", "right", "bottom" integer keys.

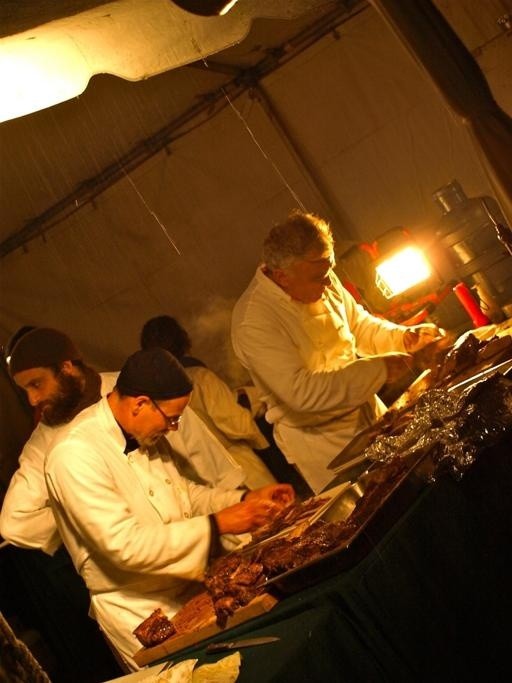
[
  {"left": 114, "top": 345, "right": 193, "bottom": 400},
  {"left": 9, "top": 327, "right": 84, "bottom": 377}
]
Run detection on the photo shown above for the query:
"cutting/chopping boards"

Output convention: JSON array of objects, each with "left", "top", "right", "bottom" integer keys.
[
  {"left": 132, "top": 519, "right": 311, "bottom": 669},
  {"left": 438, "top": 347, "right": 509, "bottom": 393}
]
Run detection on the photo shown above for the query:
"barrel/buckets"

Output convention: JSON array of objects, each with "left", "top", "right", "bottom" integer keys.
[{"left": 430, "top": 177, "right": 512, "bottom": 323}]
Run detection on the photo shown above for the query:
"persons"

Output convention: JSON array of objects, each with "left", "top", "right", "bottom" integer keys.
[
  {"left": 139, "top": 315, "right": 278, "bottom": 554},
  {"left": 43, "top": 346, "right": 295, "bottom": 673},
  {"left": 230, "top": 212, "right": 443, "bottom": 496},
  {"left": 1, "top": 324, "right": 254, "bottom": 554}
]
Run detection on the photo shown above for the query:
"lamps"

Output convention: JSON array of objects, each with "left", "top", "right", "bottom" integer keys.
[{"left": 374, "top": 245, "right": 432, "bottom": 299}]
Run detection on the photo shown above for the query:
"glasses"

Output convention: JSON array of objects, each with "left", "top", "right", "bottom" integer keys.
[{"left": 152, "top": 401, "right": 182, "bottom": 427}]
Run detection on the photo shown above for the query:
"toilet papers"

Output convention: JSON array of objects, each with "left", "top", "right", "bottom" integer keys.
[{"left": 470, "top": 282, "right": 504, "bottom": 324}]
